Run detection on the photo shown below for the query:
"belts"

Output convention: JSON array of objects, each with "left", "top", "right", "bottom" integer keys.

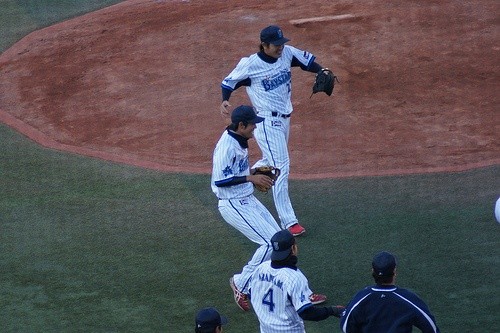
[{"left": 272, "top": 112, "right": 290, "bottom": 117}]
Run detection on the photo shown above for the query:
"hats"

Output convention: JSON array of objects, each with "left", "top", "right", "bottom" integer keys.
[
  {"left": 271, "top": 229, "right": 294, "bottom": 260},
  {"left": 195, "top": 308, "right": 228, "bottom": 329},
  {"left": 371, "top": 251, "right": 398, "bottom": 274},
  {"left": 230, "top": 105, "right": 265, "bottom": 124},
  {"left": 260, "top": 24, "right": 291, "bottom": 46}
]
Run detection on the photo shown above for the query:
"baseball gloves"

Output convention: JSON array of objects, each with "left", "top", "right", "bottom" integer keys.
[
  {"left": 311, "top": 68, "right": 335, "bottom": 96},
  {"left": 253, "top": 166, "right": 280, "bottom": 192}
]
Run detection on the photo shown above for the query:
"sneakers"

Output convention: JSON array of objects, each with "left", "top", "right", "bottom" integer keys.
[
  {"left": 229, "top": 277, "right": 250, "bottom": 313},
  {"left": 287, "top": 223, "right": 306, "bottom": 236},
  {"left": 308, "top": 294, "right": 327, "bottom": 304}
]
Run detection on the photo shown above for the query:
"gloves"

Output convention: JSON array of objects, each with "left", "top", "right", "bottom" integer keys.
[{"left": 329, "top": 305, "right": 346, "bottom": 318}]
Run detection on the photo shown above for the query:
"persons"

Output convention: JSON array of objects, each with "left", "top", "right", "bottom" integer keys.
[
  {"left": 194, "top": 307, "right": 228, "bottom": 333},
  {"left": 210, "top": 105, "right": 328, "bottom": 312},
  {"left": 250, "top": 229, "right": 345, "bottom": 333},
  {"left": 340, "top": 252, "right": 441, "bottom": 333},
  {"left": 220, "top": 25, "right": 339, "bottom": 236}
]
